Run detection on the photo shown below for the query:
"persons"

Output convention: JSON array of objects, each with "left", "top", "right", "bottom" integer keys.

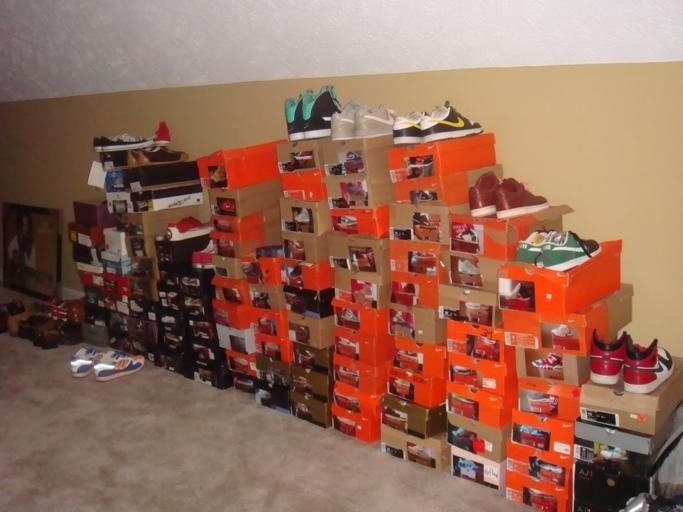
[{"left": 6, "top": 211, "right": 36, "bottom": 271}]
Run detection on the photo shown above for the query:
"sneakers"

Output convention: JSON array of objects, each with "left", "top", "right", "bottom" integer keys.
[
  {"left": 420, "top": 99, "right": 482, "bottom": 143},
  {"left": 152, "top": 122, "right": 170, "bottom": 147},
  {"left": 589, "top": 327, "right": 628, "bottom": 386},
  {"left": 330, "top": 100, "right": 361, "bottom": 141},
  {"left": 300, "top": 86, "right": 344, "bottom": 139},
  {"left": 69, "top": 347, "right": 105, "bottom": 377},
  {"left": 92, "top": 133, "right": 188, "bottom": 171},
  {"left": 619, "top": 335, "right": 673, "bottom": 394},
  {"left": 166, "top": 215, "right": 213, "bottom": 242},
  {"left": 93, "top": 349, "right": 144, "bottom": 382},
  {"left": 515, "top": 230, "right": 552, "bottom": 264},
  {"left": 283, "top": 94, "right": 303, "bottom": 142},
  {"left": 103, "top": 277, "right": 210, "bottom": 359},
  {"left": 353, "top": 104, "right": 397, "bottom": 140},
  {"left": 189, "top": 240, "right": 214, "bottom": 269},
  {"left": 505, "top": 284, "right": 577, "bottom": 512},
  {"left": 496, "top": 177, "right": 547, "bottom": 218},
  {"left": 0, "top": 296, "right": 83, "bottom": 350},
  {"left": 541, "top": 231, "right": 601, "bottom": 274},
  {"left": 391, "top": 110, "right": 430, "bottom": 145},
  {"left": 466, "top": 170, "right": 500, "bottom": 219}
]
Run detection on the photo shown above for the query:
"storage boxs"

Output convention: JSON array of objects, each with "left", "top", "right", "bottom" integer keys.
[
  {"left": 195, "top": 131, "right": 573, "bottom": 492},
  {"left": 498, "top": 238, "right": 680, "bottom": 512},
  {"left": 67, "top": 160, "right": 232, "bottom": 389}
]
